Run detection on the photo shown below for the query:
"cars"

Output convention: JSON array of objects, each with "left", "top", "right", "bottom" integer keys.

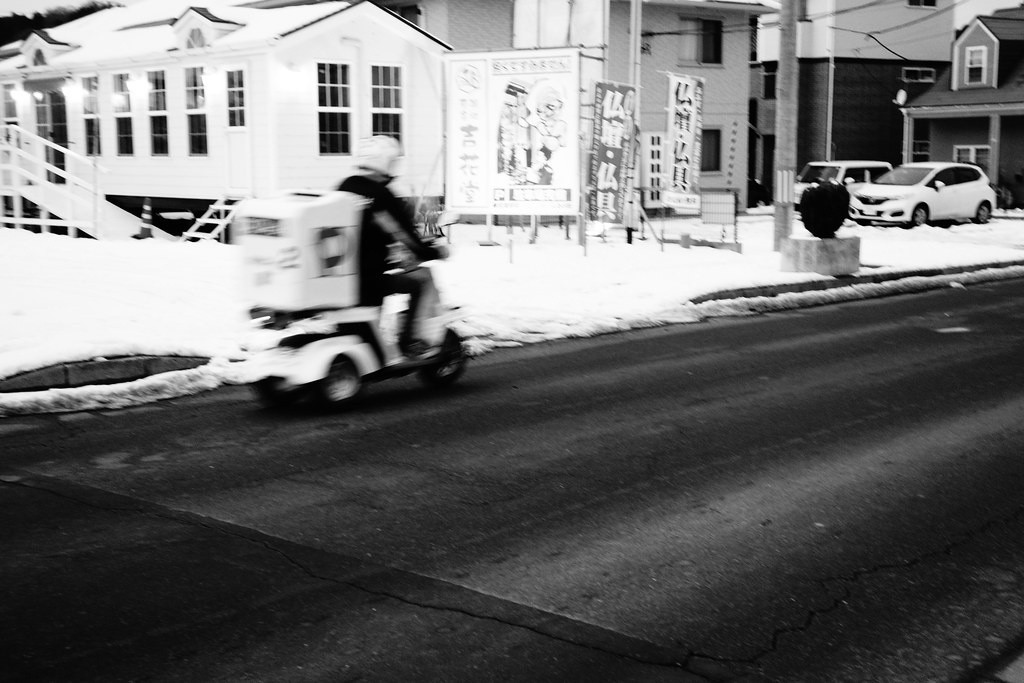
[{"left": 847, "top": 161, "right": 996, "bottom": 229}]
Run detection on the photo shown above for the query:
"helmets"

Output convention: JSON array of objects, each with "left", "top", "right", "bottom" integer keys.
[{"left": 356, "top": 134, "right": 403, "bottom": 177}]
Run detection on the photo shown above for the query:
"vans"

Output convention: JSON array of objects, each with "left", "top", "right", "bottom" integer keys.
[{"left": 792, "top": 160, "right": 896, "bottom": 218}]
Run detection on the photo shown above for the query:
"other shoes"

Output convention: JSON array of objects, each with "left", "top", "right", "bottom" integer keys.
[{"left": 401, "top": 340, "right": 430, "bottom": 357}]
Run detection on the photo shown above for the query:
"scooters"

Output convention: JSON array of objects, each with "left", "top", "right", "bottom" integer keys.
[{"left": 211, "top": 185, "right": 468, "bottom": 413}]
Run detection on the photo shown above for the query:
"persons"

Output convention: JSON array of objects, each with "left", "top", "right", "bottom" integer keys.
[{"left": 332, "top": 133, "right": 451, "bottom": 362}]
